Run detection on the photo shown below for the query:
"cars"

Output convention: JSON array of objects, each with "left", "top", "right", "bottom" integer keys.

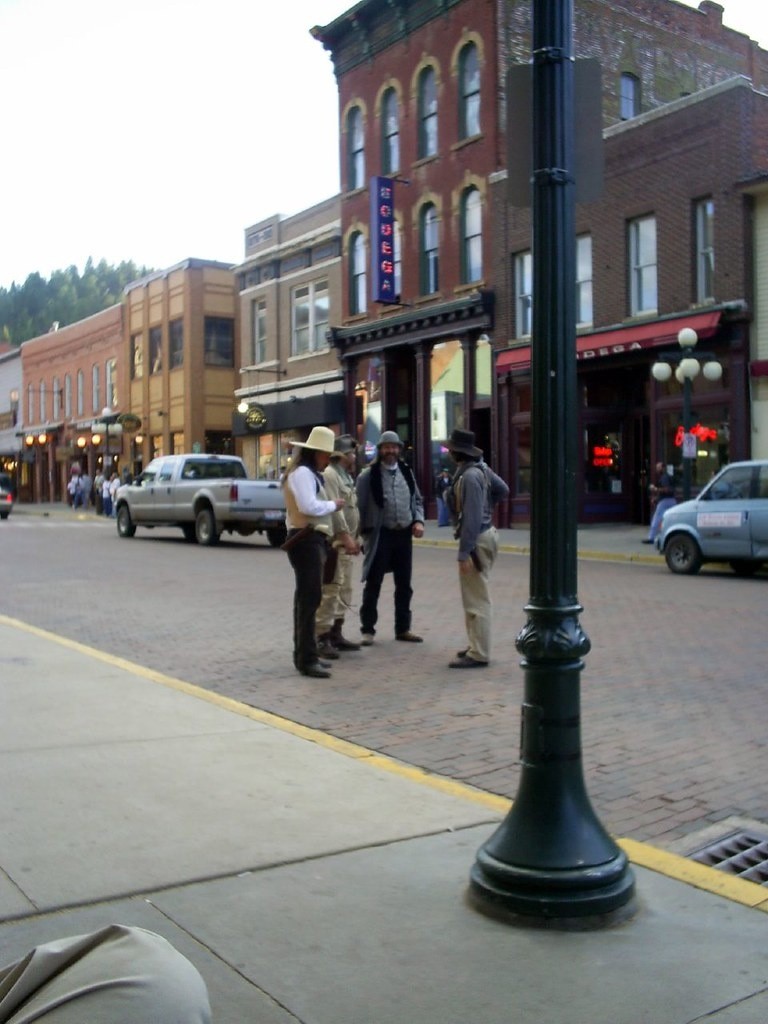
[{"left": 0, "top": 486, "right": 14, "bottom": 518}]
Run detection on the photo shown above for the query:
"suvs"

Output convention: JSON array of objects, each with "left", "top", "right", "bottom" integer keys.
[{"left": 655, "top": 461, "right": 768, "bottom": 574}]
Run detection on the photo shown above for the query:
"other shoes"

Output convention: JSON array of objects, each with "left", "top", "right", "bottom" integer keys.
[
  {"left": 449, "top": 655, "right": 488, "bottom": 668},
  {"left": 395, "top": 631, "right": 423, "bottom": 641},
  {"left": 360, "top": 633, "right": 373, "bottom": 644},
  {"left": 300, "top": 664, "right": 332, "bottom": 677},
  {"left": 458, "top": 650, "right": 468, "bottom": 657},
  {"left": 313, "top": 657, "right": 331, "bottom": 667}
]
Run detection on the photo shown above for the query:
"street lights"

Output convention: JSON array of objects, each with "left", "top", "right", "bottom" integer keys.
[
  {"left": 91, "top": 407, "right": 121, "bottom": 478},
  {"left": 651, "top": 327, "right": 724, "bottom": 502}
]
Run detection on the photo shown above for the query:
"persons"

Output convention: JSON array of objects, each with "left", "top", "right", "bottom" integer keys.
[
  {"left": 436, "top": 428, "right": 510, "bottom": 668},
  {"left": 281, "top": 425, "right": 366, "bottom": 679},
  {"left": 642, "top": 462, "right": 678, "bottom": 544},
  {"left": 67, "top": 466, "right": 133, "bottom": 517},
  {"left": 356, "top": 431, "right": 426, "bottom": 646},
  {"left": 1, "top": 924, "right": 214, "bottom": 1024}
]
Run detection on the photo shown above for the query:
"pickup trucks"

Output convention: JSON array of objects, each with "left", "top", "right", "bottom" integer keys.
[{"left": 114, "top": 455, "right": 290, "bottom": 546}]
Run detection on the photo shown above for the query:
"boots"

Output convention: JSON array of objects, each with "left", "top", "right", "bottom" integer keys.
[
  {"left": 330, "top": 619, "right": 359, "bottom": 648},
  {"left": 316, "top": 632, "right": 339, "bottom": 659}
]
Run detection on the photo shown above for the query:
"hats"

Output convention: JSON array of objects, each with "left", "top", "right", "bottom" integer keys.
[
  {"left": 439, "top": 429, "right": 483, "bottom": 457},
  {"left": 334, "top": 434, "right": 357, "bottom": 454},
  {"left": 289, "top": 427, "right": 348, "bottom": 459},
  {"left": 376, "top": 431, "right": 404, "bottom": 448}
]
